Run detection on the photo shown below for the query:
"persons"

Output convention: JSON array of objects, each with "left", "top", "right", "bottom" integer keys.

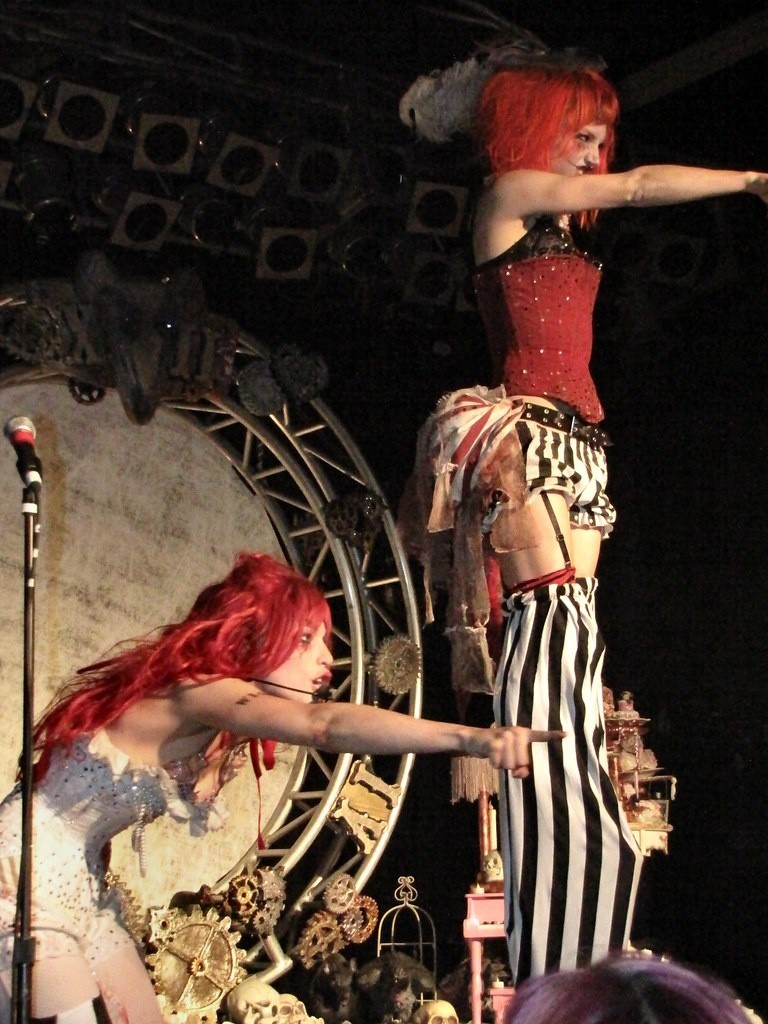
[
  {"left": 417, "top": 59, "right": 768, "bottom": 988},
  {"left": 0, "top": 555, "right": 567, "bottom": 1024},
  {"left": 504, "top": 955, "right": 752, "bottom": 1024}
]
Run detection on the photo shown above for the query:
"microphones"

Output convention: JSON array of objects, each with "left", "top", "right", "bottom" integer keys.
[{"left": 2, "top": 416, "right": 44, "bottom": 493}]
